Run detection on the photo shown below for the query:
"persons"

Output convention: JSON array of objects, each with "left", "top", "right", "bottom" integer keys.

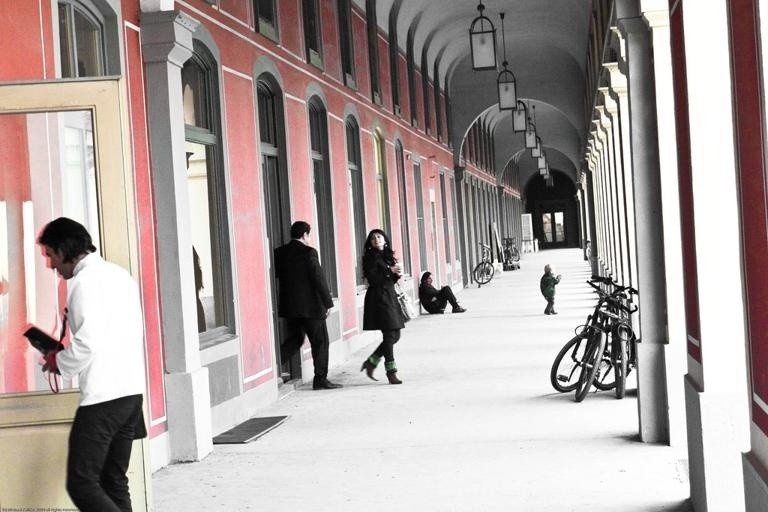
[
  {"left": 361, "top": 229, "right": 411, "bottom": 384},
  {"left": 36, "top": 217, "right": 147, "bottom": 512},
  {"left": 193, "top": 246, "right": 207, "bottom": 332},
  {"left": 274, "top": 221, "right": 344, "bottom": 390},
  {"left": 540, "top": 264, "right": 562, "bottom": 315},
  {"left": 419, "top": 272, "right": 466, "bottom": 314}
]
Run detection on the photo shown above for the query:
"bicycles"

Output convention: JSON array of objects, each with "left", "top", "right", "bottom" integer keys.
[
  {"left": 551, "top": 274, "right": 638, "bottom": 404},
  {"left": 474, "top": 243, "right": 494, "bottom": 284},
  {"left": 502, "top": 238, "right": 520, "bottom": 264}
]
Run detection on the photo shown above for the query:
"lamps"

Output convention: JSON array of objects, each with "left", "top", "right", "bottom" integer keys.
[{"left": 468, "top": 0, "right": 555, "bottom": 189}]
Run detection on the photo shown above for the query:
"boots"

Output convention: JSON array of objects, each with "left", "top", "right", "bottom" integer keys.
[{"left": 384, "top": 367, "right": 404, "bottom": 385}]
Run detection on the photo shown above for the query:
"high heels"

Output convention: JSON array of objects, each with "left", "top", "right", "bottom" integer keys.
[
  {"left": 359, "top": 358, "right": 379, "bottom": 382},
  {"left": 310, "top": 372, "right": 346, "bottom": 391}
]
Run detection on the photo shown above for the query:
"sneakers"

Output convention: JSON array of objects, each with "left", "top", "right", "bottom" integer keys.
[
  {"left": 451, "top": 307, "right": 466, "bottom": 313},
  {"left": 545, "top": 309, "right": 557, "bottom": 315}
]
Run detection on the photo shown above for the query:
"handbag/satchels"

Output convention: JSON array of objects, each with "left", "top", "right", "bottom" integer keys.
[{"left": 397, "top": 291, "right": 410, "bottom": 321}]
